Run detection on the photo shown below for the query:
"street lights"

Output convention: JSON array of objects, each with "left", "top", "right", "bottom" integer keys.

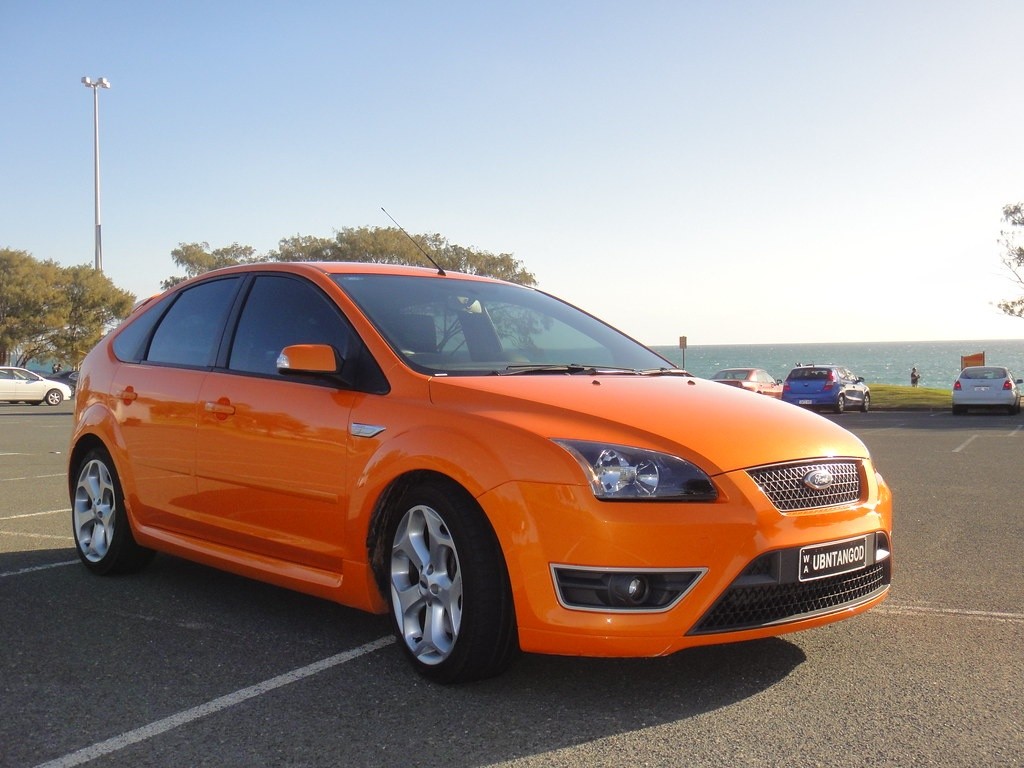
[{"left": 81, "top": 77, "right": 111, "bottom": 274}]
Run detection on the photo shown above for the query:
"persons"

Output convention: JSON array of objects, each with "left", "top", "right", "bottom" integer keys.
[
  {"left": 52, "top": 364, "right": 62, "bottom": 373},
  {"left": 911, "top": 368, "right": 920, "bottom": 387}
]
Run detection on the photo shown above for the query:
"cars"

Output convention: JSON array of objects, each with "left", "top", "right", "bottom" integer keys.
[
  {"left": 952, "top": 365, "right": 1024, "bottom": 416},
  {"left": 68, "top": 209, "right": 892, "bottom": 683},
  {"left": 0, "top": 366, "right": 71, "bottom": 406},
  {"left": 782, "top": 363, "right": 871, "bottom": 414},
  {"left": 28, "top": 368, "right": 52, "bottom": 380},
  {"left": 43, "top": 371, "right": 79, "bottom": 396},
  {"left": 710, "top": 368, "right": 783, "bottom": 399}
]
